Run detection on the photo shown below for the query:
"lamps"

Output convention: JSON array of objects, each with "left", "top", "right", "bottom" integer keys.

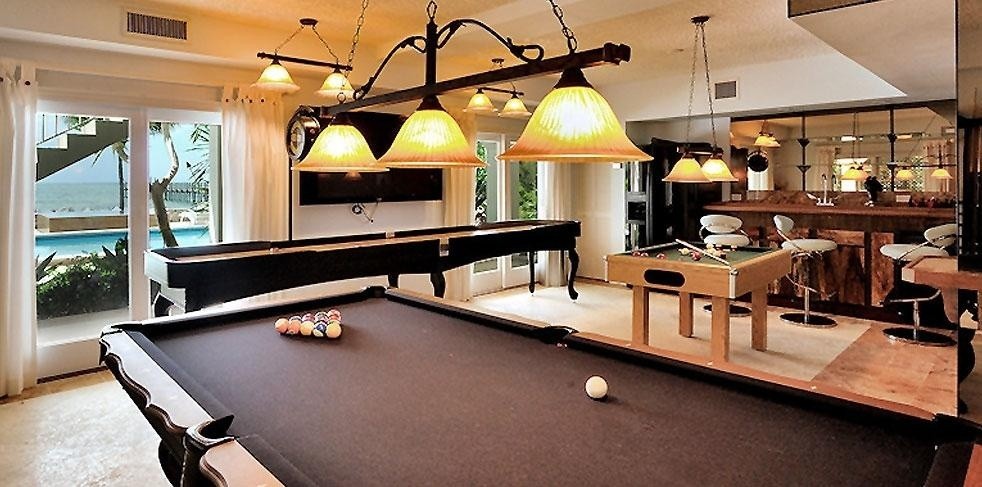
[
  {"left": 661, "top": 12, "right": 740, "bottom": 186},
  {"left": 253, "top": 17, "right": 365, "bottom": 103},
  {"left": 753, "top": 116, "right": 781, "bottom": 148},
  {"left": 895, "top": 112, "right": 955, "bottom": 187},
  {"left": 462, "top": 55, "right": 534, "bottom": 120},
  {"left": 840, "top": 108, "right": 867, "bottom": 183},
  {"left": 287, "top": 0, "right": 657, "bottom": 173},
  {"left": 341, "top": 169, "right": 362, "bottom": 181}
]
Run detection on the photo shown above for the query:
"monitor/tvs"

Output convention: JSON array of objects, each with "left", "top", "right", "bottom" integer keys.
[{"left": 299, "top": 106, "right": 443, "bottom": 207}]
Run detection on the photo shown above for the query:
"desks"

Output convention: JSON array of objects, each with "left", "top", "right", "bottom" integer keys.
[
  {"left": 140, "top": 215, "right": 582, "bottom": 316},
  {"left": 99, "top": 282, "right": 982, "bottom": 487},
  {"left": 602, "top": 240, "right": 794, "bottom": 363}
]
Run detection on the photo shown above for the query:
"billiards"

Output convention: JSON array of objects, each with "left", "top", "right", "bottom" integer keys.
[
  {"left": 633, "top": 251, "right": 647, "bottom": 257},
  {"left": 703, "top": 242, "right": 737, "bottom": 260},
  {"left": 586, "top": 376, "right": 607, "bottom": 398},
  {"left": 274, "top": 309, "right": 342, "bottom": 337},
  {"left": 680, "top": 247, "right": 690, "bottom": 256},
  {"left": 657, "top": 253, "right": 665, "bottom": 260},
  {"left": 691, "top": 251, "right": 701, "bottom": 261}
]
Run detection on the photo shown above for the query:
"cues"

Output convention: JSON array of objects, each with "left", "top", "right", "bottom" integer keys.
[{"left": 676, "top": 239, "right": 730, "bottom": 267}]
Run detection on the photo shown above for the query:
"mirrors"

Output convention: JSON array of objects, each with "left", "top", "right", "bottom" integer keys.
[{"left": 727, "top": 98, "right": 959, "bottom": 194}]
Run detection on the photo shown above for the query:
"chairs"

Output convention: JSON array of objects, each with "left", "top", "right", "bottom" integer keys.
[
  {"left": 697, "top": 212, "right": 755, "bottom": 318},
  {"left": 772, "top": 214, "right": 841, "bottom": 328},
  {"left": 877, "top": 215, "right": 957, "bottom": 349}
]
